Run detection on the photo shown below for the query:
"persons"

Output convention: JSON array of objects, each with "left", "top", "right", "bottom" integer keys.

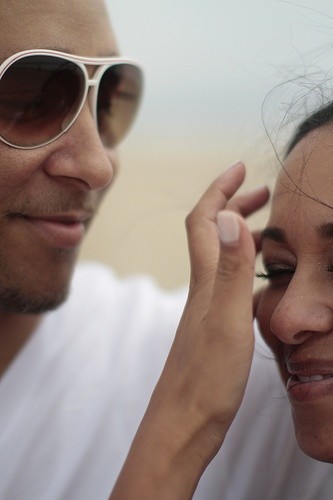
[
  {"left": 109, "top": 102, "right": 333, "bottom": 500},
  {"left": 0, "top": 0, "right": 333, "bottom": 500}
]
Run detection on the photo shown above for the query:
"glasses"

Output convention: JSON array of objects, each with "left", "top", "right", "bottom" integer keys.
[{"left": 0, "top": 48, "right": 145, "bottom": 150}]
